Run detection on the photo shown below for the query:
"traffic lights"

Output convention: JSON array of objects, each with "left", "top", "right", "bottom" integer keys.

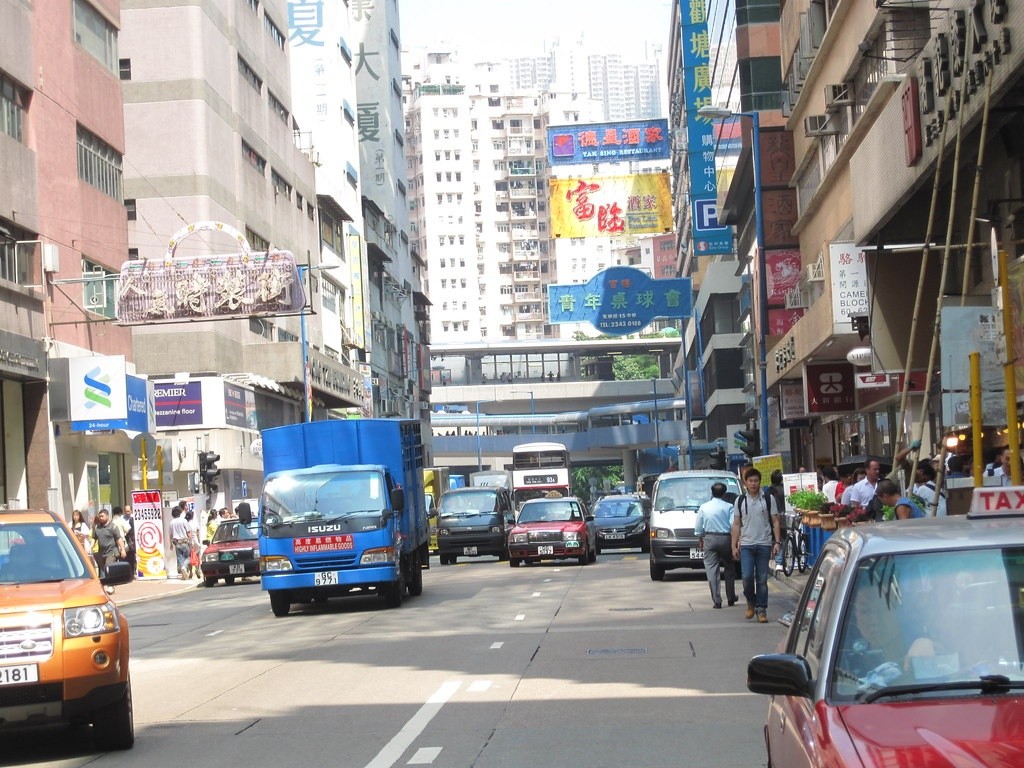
[{"left": 204, "top": 452, "right": 223, "bottom": 483}]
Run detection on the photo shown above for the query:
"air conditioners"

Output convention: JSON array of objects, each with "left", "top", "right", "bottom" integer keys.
[
  {"left": 537, "top": 190, "right": 543, "bottom": 196},
  {"left": 784, "top": 293, "right": 808, "bottom": 309},
  {"left": 372, "top": 378, "right": 382, "bottom": 387},
  {"left": 381, "top": 399, "right": 392, "bottom": 411},
  {"left": 803, "top": 113, "right": 840, "bottom": 137},
  {"left": 420, "top": 402, "right": 429, "bottom": 410},
  {"left": 393, "top": 388, "right": 414, "bottom": 402},
  {"left": 806, "top": 264, "right": 824, "bottom": 282},
  {"left": 536, "top": 171, "right": 542, "bottom": 177},
  {"left": 824, "top": 82, "right": 855, "bottom": 108}
]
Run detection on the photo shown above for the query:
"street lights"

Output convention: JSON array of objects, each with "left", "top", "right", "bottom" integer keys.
[
  {"left": 653, "top": 310, "right": 708, "bottom": 471},
  {"left": 510, "top": 390, "right": 536, "bottom": 435},
  {"left": 476, "top": 400, "right": 497, "bottom": 472},
  {"left": 301, "top": 265, "right": 341, "bottom": 421},
  {"left": 694, "top": 103, "right": 771, "bottom": 456}
]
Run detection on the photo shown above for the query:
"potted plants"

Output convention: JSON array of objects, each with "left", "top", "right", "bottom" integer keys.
[{"left": 786, "top": 493, "right": 836, "bottom": 529}]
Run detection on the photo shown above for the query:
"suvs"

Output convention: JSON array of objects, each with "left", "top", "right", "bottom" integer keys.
[
  {"left": 591, "top": 495, "right": 653, "bottom": 552},
  {"left": 0, "top": 506, "right": 142, "bottom": 752}
]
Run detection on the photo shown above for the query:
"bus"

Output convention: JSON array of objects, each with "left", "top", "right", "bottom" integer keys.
[{"left": 512, "top": 442, "right": 572, "bottom": 522}]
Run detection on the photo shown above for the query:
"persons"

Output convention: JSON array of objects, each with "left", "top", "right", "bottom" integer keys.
[
  {"left": 169, "top": 501, "right": 239, "bottom": 580},
  {"left": 843, "top": 569, "right": 949, "bottom": 673},
  {"left": 694, "top": 469, "right": 782, "bottom": 624},
  {"left": 72, "top": 505, "right": 136, "bottom": 577},
  {"left": 799, "top": 439, "right": 1024, "bottom": 521}
]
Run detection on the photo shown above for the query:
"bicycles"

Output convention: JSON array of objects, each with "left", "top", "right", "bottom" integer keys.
[{"left": 778, "top": 510, "right": 811, "bottom": 577}]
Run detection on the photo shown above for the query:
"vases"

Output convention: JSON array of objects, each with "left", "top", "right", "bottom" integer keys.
[{"left": 834, "top": 517, "right": 870, "bottom": 530}]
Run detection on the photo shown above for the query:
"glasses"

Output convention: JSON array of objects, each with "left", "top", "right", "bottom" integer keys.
[{"left": 1003, "top": 456, "right": 1009, "bottom": 461}]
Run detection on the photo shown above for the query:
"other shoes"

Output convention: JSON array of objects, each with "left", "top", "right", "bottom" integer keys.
[
  {"left": 181, "top": 565, "right": 189, "bottom": 579},
  {"left": 728, "top": 596, "right": 738, "bottom": 606},
  {"left": 195, "top": 570, "right": 200, "bottom": 578},
  {"left": 189, "top": 573, "right": 192, "bottom": 578},
  {"left": 713, "top": 602, "right": 721, "bottom": 608}
]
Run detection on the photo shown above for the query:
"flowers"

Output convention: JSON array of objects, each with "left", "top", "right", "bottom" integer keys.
[{"left": 830, "top": 505, "right": 871, "bottom": 526}]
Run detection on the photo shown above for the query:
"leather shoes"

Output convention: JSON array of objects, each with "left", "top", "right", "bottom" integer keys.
[
  {"left": 745, "top": 607, "right": 754, "bottom": 618},
  {"left": 757, "top": 615, "right": 768, "bottom": 623}
]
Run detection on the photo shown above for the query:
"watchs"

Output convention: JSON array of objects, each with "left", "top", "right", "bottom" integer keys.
[{"left": 774, "top": 542, "right": 781, "bottom": 544}]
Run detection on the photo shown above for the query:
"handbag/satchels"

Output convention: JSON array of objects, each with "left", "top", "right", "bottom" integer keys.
[
  {"left": 190, "top": 546, "right": 200, "bottom": 566},
  {"left": 84, "top": 537, "right": 92, "bottom": 554},
  {"left": 114, "top": 547, "right": 121, "bottom": 558}
]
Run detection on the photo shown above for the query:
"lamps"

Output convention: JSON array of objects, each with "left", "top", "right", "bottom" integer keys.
[
  {"left": 858, "top": 39, "right": 923, "bottom": 63},
  {"left": 872, "top": 0, "right": 950, "bottom": 11}
]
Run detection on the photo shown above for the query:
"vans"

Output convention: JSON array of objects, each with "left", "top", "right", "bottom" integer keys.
[
  {"left": 436, "top": 486, "right": 512, "bottom": 565},
  {"left": 650, "top": 469, "right": 747, "bottom": 581}
]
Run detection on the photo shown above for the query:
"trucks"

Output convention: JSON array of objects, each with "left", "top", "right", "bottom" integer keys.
[
  {"left": 257, "top": 418, "right": 425, "bottom": 617},
  {"left": 423, "top": 465, "right": 450, "bottom": 554},
  {"left": 449, "top": 471, "right": 514, "bottom": 499}
]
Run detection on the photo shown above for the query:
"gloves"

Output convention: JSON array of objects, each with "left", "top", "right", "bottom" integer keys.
[{"left": 909, "top": 439, "right": 922, "bottom": 449}]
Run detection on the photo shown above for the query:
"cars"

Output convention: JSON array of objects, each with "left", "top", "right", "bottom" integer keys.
[
  {"left": 201, "top": 516, "right": 260, "bottom": 587},
  {"left": 747, "top": 482, "right": 1024, "bottom": 768},
  {"left": 508, "top": 491, "right": 597, "bottom": 567}
]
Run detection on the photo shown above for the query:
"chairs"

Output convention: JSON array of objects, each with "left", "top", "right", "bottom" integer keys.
[{"left": 1, "top": 546, "right": 34, "bottom": 583}]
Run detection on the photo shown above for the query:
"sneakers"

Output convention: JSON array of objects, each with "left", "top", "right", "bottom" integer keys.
[
  {"left": 776, "top": 564, "right": 789, "bottom": 572},
  {"left": 793, "top": 562, "right": 803, "bottom": 569},
  {"left": 106, "top": 586, "right": 115, "bottom": 595}
]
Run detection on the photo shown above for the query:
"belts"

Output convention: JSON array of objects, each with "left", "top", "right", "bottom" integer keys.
[{"left": 706, "top": 532, "right": 730, "bottom": 536}]
